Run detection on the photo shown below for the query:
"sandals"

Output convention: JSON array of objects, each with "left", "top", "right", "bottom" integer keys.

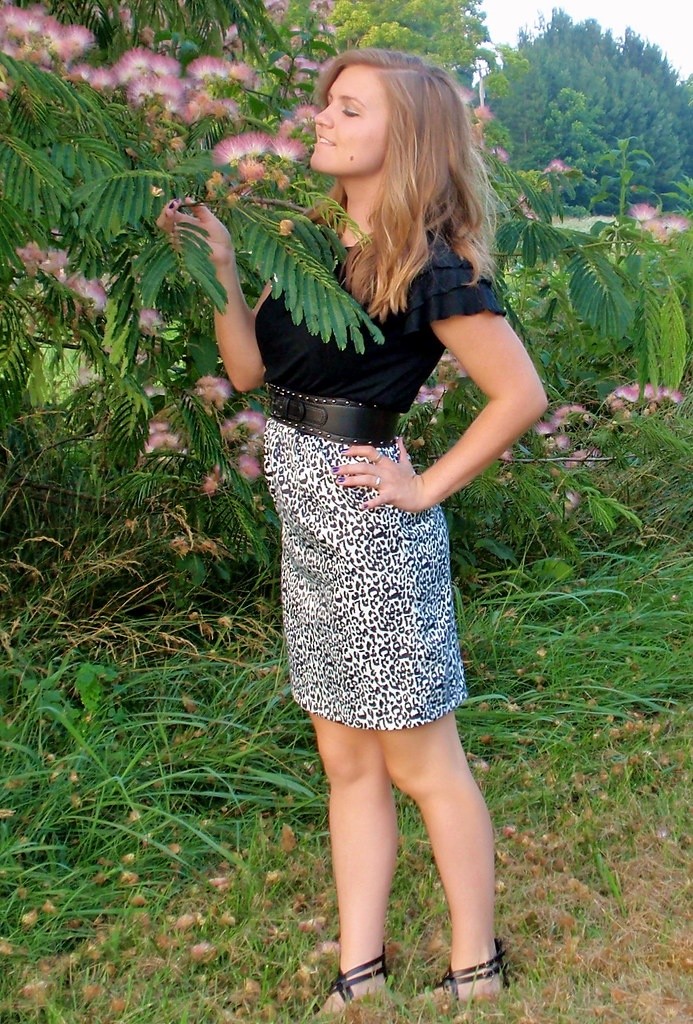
[
  {"left": 311, "top": 945, "right": 395, "bottom": 1019},
  {"left": 408, "top": 938, "right": 511, "bottom": 1006}
]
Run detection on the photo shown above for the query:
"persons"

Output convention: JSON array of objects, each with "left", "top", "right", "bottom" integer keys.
[{"left": 156, "top": 48, "right": 548, "bottom": 1021}]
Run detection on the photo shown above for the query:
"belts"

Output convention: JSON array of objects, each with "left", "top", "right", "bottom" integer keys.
[{"left": 262, "top": 382, "right": 400, "bottom": 448}]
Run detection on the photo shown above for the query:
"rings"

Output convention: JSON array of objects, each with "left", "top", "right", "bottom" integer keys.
[
  {"left": 374, "top": 476, "right": 381, "bottom": 488},
  {"left": 373, "top": 452, "right": 383, "bottom": 463}
]
